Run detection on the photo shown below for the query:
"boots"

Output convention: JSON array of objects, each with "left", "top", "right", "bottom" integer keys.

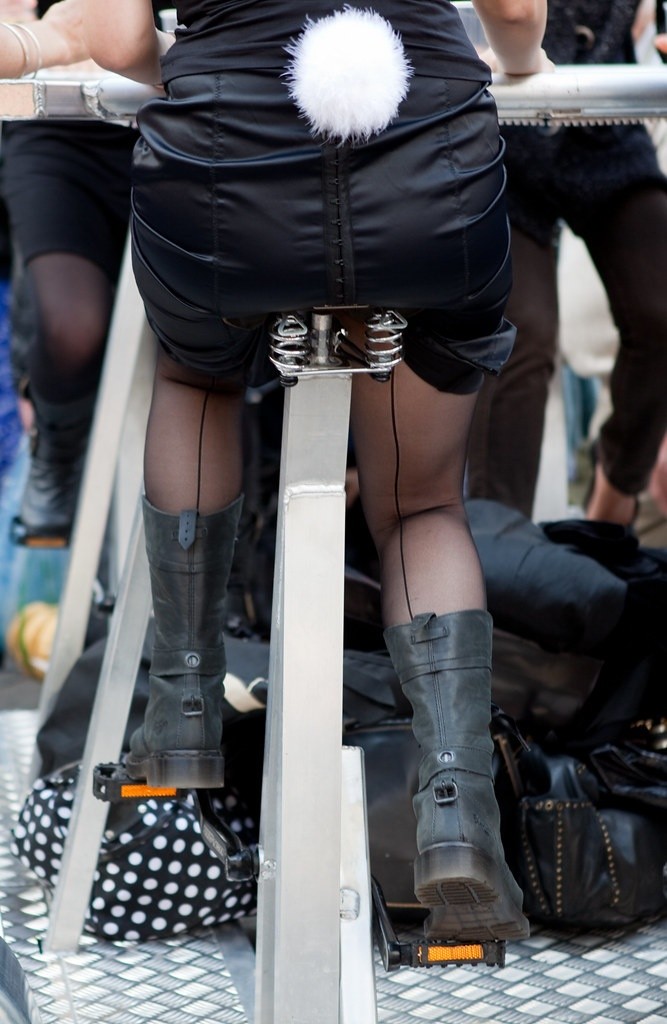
[
  {"left": 125, "top": 491, "right": 246, "bottom": 789},
  {"left": 382, "top": 608, "right": 531, "bottom": 942}
]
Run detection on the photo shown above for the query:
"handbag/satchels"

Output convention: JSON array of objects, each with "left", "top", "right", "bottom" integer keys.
[
  {"left": 42, "top": 458, "right": 665, "bottom": 931},
  {"left": 9, "top": 758, "right": 261, "bottom": 942}
]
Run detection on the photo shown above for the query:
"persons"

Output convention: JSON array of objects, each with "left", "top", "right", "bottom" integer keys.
[
  {"left": 0, "top": 0, "right": 127, "bottom": 673},
  {"left": 460, "top": 1, "right": 666, "bottom": 534},
  {"left": 81, "top": 0, "right": 558, "bottom": 936}
]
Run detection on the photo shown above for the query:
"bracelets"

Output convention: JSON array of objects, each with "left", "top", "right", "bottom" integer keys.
[
  {"left": 154, "top": 30, "right": 176, "bottom": 89},
  {"left": 10, "top": 22, "right": 42, "bottom": 79},
  {"left": 0, "top": 23, "right": 29, "bottom": 79}
]
